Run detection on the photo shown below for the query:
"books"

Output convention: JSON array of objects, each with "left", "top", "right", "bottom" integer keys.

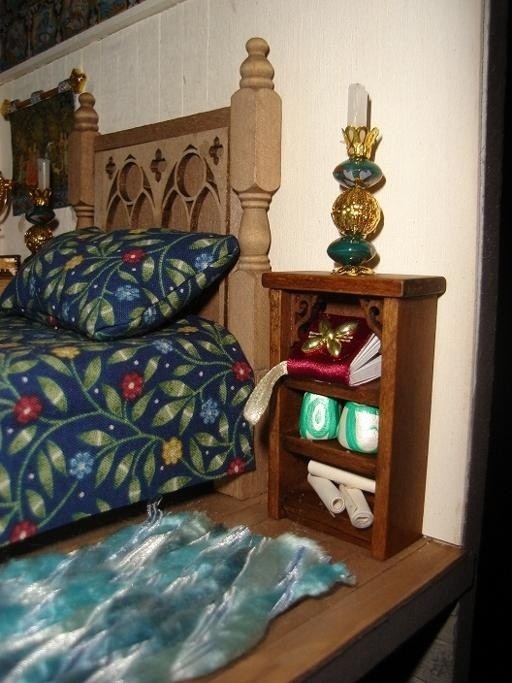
[{"left": 289, "top": 316, "right": 385, "bottom": 387}]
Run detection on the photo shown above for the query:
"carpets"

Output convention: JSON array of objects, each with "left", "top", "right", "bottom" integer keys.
[{"left": 0, "top": 507, "right": 359, "bottom": 683}]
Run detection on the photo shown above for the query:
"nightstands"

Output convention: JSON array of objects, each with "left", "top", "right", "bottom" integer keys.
[{"left": 260, "top": 264, "right": 446, "bottom": 560}]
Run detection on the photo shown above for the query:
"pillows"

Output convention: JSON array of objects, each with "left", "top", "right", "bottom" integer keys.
[{"left": 2, "top": 223, "right": 241, "bottom": 338}]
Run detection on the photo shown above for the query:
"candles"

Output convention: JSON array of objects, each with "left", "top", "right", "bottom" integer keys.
[
  {"left": 347, "top": 81, "right": 368, "bottom": 131},
  {"left": 37, "top": 156, "right": 51, "bottom": 191}
]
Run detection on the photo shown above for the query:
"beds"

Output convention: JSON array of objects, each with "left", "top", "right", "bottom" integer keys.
[{"left": 0, "top": 37, "right": 283, "bottom": 550}]
